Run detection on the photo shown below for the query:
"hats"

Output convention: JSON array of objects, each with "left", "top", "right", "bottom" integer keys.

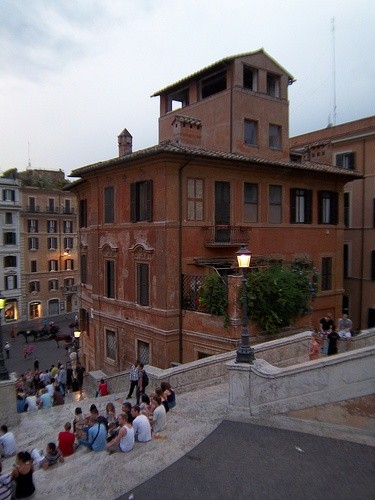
[
  {"left": 84, "top": 412, "right": 91, "bottom": 419},
  {"left": 60, "top": 365, "right": 64, "bottom": 368}
]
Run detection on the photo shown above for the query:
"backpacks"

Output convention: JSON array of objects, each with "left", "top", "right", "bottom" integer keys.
[{"left": 140, "top": 371, "right": 149, "bottom": 387}]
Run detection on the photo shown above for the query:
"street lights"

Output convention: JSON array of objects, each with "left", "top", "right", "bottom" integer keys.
[
  {"left": 235, "top": 244, "right": 256, "bottom": 364},
  {"left": 0, "top": 290, "right": 11, "bottom": 380},
  {"left": 73, "top": 328, "right": 81, "bottom": 370}
]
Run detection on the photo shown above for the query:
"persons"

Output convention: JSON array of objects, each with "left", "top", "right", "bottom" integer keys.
[
  {"left": 131, "top": 405, "right": 151, "bottom": 443},
  {"left": 147, "top": 395, "right": 167, "bottom": 432},
  {"left": 77, "top": 414, "right": 107, "bottom": 450},
  {"left": 12, "top": 451, "right": 37, "bottom": 500},
  {"left": 103, "top": 412, "right": 135, "bottom": 452},
  {"left": 3, "top": 341, "right": 10, "bottom": 359},
  {"left": 0, "top": 462, "right": 16, "bottom": 500},
  {"left": 57, "top": 421, "right": 81, "bottom": 456},
  {"left": 72, "top": 380, "right": 133, "bottom": 441},
  {"left": 126, "top": 359, "right": 176, "bottom": 413},
  {"left": 0, "top": 425, "right": 18, "bottom": 458},
  {"left": 11, "top": 315, "right": 87, "bottom": 413},
  {"left": 306, "top": 313, "right": 353, "bottom": 359},
  {"left": 31, "top": 442, "right": 65, "bottom": 470}
]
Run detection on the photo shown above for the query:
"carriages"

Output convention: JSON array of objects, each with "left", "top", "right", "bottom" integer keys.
[
  {"left": 16, "top": 325, "right": 59, "bottom": 344},
  {"left": 48, "top": 321, "right": 79, "bottom": 351}
]
